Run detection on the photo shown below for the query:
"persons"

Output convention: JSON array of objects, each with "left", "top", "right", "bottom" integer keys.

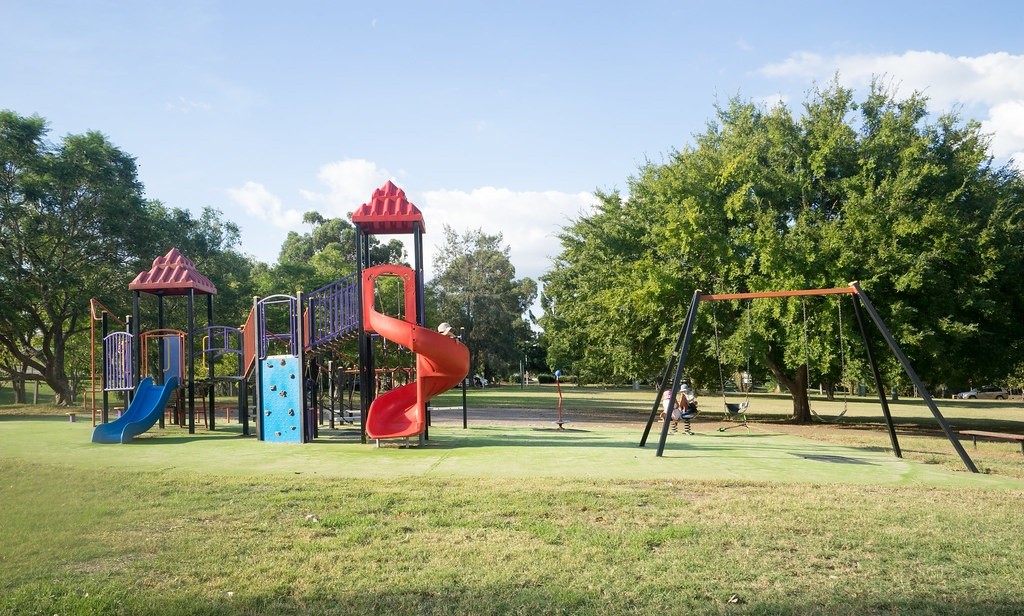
[
  {"left": 474, "top": 377, "right": 483, "bottom": 387},
  {"left": 661, "top": 390, "right": 682, "bottom": 421},
  {"left": 438, "top": 322, "right": 462, "bottom": 341},
  {"left": 383, "top": 367, "right": 392, "bottom": 391},
  {"left": 679, "top": 383, "right": 698, "bottom": 420}
]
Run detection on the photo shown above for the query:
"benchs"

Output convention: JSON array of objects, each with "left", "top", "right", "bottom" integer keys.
[{"left": 165, "top": 403, "right": 239, "bottom": 424}]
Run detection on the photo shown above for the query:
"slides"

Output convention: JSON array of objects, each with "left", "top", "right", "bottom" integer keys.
[
  {"left": 363, "top": 308, "right": 470, "bottom": 438},
  {"left": 92, "top": 376, "right": 180, "bottom": 444}
]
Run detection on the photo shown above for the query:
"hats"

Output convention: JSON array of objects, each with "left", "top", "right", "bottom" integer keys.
[
  {"left": 680, "top": 384, "right": 690, "bottom": 391},
  {"left": 660, "top": 390, "right": 672, "bottom": 400},
  {"left": 438, "top": 323, "right": 452, "bottom": 335}
]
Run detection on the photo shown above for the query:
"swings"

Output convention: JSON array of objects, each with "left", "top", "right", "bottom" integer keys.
[
  {"left": 711, "top": 299, "right": 752, "bottom": 414},
  {"left": 800, "top": 295, "right": 848, "bottom": 421}
]
